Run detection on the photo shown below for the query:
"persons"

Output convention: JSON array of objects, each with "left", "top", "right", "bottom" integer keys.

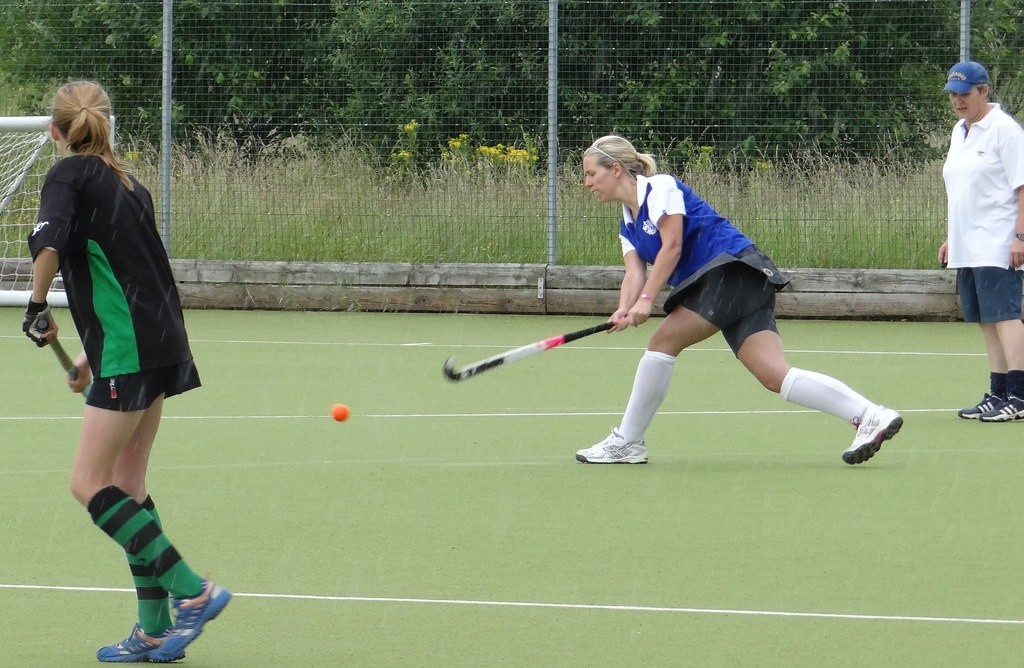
[
  {"left": 21, "top": 82, "right": 232, "bottom": 662},
  {"left": 575, "top": 135, "right": 903, "bottom": 465},
  {"left": 938, "top": 62, "right": 1024, "bottom": 421}
]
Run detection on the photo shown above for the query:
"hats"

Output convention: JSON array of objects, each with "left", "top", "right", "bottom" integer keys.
[{"left": 942, "top": 62, "right": 990, "bottom": 94}]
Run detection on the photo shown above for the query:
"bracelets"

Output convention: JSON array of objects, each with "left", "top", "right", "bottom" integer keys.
[{"left": 641, "top": 292, "right": 651, "bottom": 300}]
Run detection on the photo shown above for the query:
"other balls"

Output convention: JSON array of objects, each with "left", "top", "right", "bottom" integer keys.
[{"left": 330, "top": 403, "right": 351, "bottom": 423}]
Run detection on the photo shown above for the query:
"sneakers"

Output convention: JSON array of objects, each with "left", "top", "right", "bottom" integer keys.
[
  {"left": 842, "top": 405, "right": 903, "bottom": 466},
  {"left": 96, "top": 623, "right": 186, "bottom": 663},
  {"left": 159, "top": 577, "right": 231, "bottom": 655},
  {"left": 958, "top": 392, "right": 1024, "bottom": 423},
  {"left": 575, "top": 425, "right": 649, "bottom": 464}
]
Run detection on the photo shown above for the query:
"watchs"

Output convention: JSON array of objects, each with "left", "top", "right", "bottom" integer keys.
[{"left": 1016, "top": 233, "right": 1023, "bottom": 241}]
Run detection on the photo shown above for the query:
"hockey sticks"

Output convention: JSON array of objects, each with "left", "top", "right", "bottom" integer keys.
[
  {"left": 442, "top": 321, "right": 616, "bottom": 382},
  {"left": 37, "top": 319, "right": 92, "bottom": 401}
]
